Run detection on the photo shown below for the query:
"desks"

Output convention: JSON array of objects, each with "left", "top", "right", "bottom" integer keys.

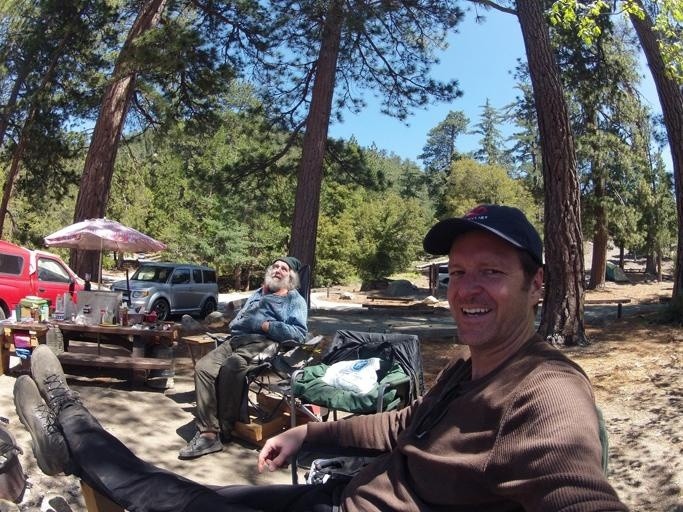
[{"left": 0, "top": 313, "right": 180, "bottom": 374}]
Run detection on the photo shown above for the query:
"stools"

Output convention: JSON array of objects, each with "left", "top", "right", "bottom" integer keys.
[{"left": 182, "top": 333, "right": 232, "bottom": 365}]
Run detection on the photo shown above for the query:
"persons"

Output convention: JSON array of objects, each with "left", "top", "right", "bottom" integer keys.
[
  {"left": 14, "top": 206, "right": 632, "bottom": 511},
  {"left": 178, "top": 255, "right": 310, "bottom": 461}
]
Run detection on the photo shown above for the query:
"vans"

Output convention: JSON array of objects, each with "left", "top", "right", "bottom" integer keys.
[{"left": 110, "top": 262, "right": 218, "bottom": 322}]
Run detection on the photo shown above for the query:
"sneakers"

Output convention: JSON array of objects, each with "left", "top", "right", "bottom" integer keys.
[
  {"left": 30, "top": 344, "right": 83, "bottom": 415},
  {"left": 13, "top": 374, "right": 64, "bottom": 476},
  {"left": 180, "top": 431, "right": 224, "bottom": 458}
]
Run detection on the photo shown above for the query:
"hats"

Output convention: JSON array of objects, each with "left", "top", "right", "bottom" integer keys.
[
  {"left": 423, "top": 204, "right": 542, "bottom": 266},
  {"left": 272, "top": 257, "right": 301, "bottom": 273}
]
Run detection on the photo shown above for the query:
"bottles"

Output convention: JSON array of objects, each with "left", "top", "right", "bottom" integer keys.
[{"left": 11, "top": 292, "right": 127, "bottom": 327}]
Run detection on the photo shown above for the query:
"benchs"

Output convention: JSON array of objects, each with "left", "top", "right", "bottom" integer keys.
[
  {"left": 366, "top": 296, "right": 414, "bottom": 304},
  {"left": 12, "top": 349, "right": 175, "bottom": 385},
  {"left": 623, "top": 269, "right": 642, "bottom": 272},
  {"left": 362, "top": 301, "right": 416, "bottom": 313},
  {"left": 537, "top": 297, "right": 630, "bottom": 320}
]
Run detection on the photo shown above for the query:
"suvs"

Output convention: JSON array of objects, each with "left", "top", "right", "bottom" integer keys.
[{"left": 0, "top": 240, "right": 92, "bottom": 322}]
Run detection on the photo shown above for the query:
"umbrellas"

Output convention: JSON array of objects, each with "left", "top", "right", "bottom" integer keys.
[{"left": 45, "top": 215, "right": 167, "bottom": 287}]
